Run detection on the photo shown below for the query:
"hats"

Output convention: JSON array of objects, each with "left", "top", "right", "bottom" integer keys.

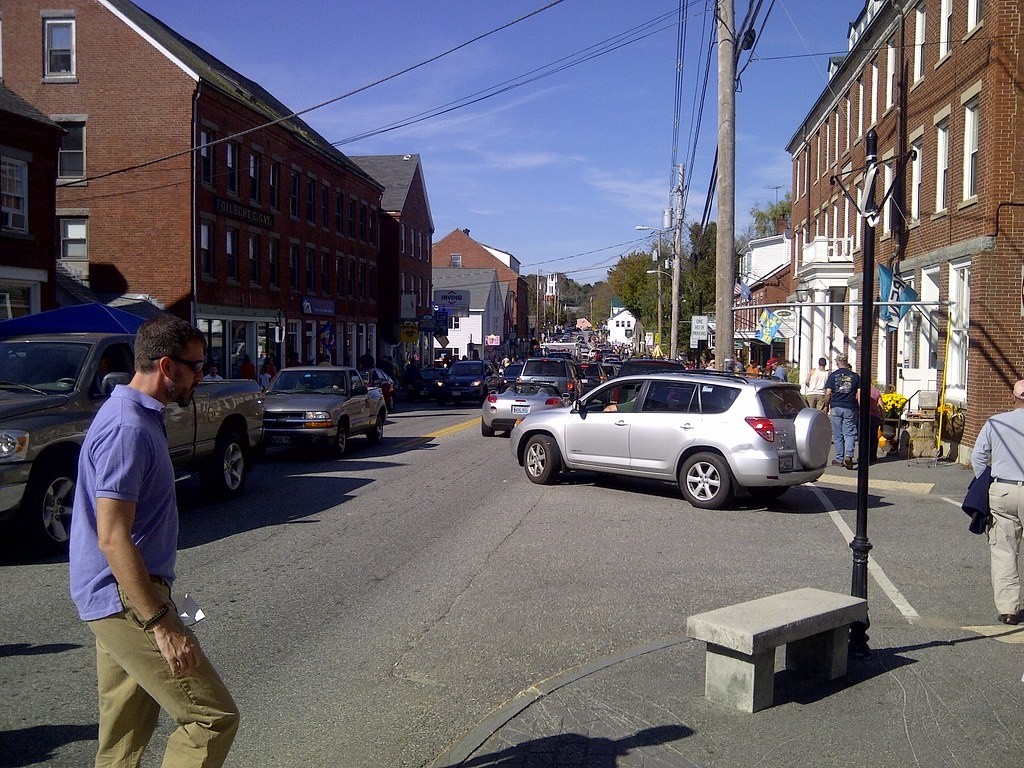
[
  {"left": 1012, "top": 379, "right": 1024, "bottom": 399},
  {"left": 834, "top": 353, "right": 848, "bottom": 361}
]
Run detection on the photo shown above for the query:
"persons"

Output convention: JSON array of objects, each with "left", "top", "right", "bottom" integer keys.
[
  {"left": 376, "top": 355, "right": 396, "bottom": 378},
  {"left": 360, "top": 349, "right": 374, "bottom": 369},
  {"left": 603, "top": 401, "right": 635, "bottom": 412},
  {"left": 688, "top": 359, "right": 715, "bottom": 371},
  {"left": 93, "top": 348, "right": 112, "bottom": 394},
  {"left": 499, "top": 354, "right": 516, "bottom": 373},
  {"left": 203, "top": 365, "right": 223, "bottom": 380},
  {"left": 289, "top": 352, "right": 332, "bottom": 388},
  {"left": 259, "top": 357, "right": 277, "bottom": 388},
  {"left": 69, "top": 314, "right": 239, "bottom": 768},
  {"left": 971, "top": 380, "right": 1024, "bottom": 625},
  {"left": 240, "top": 354, "right": 255, "bottom": 379},
  {"left": 443, "top": 354, "right": 468, "bottom": 369},
  {"left": 805, "top": 357, "right": 885, "bottom": 470},
  {"left": 406, "top": 358, "right": 417, "bottom": 384},
  {"left": 734, "top": 356, "right": 788, "bottom": 382}
]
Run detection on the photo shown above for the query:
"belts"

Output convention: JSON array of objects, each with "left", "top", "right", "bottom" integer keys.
[
  {"left": 149, "top": 575, "right": 168, "bottom": 587},
  {"left": 993, "top": 478, "right": 1024, "bottom": 486}
]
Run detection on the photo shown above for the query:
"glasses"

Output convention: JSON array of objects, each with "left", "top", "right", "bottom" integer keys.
[{"left": 148, "top": 355, "right": 204, "bottom": 373}]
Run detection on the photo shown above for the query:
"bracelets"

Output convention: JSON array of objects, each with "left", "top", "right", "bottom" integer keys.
[{"left": 144, "top": 605, "right": 169, "bottom": 630}]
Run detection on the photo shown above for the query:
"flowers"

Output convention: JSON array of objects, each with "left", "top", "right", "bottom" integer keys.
[{"left": 881, "top": 393, "right": 907, "bottom": 418}]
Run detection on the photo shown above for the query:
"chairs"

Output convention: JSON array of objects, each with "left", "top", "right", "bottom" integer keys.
[
  {"left": 505, "top": 387, "right": 515, "bottom": 393},
  {"left": 537, "top": 387, "right": 548, "bottom": 395},
  {"left": 297, "top": 374, "right": 314, "bottom": 388}
]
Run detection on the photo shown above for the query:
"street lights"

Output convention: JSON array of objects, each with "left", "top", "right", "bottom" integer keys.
[
  {"left": 634, "top": 225, "right": 662, "bottom": 359},
  {"left": 645, "top": 270, "right": 680, "bottom": 360}
]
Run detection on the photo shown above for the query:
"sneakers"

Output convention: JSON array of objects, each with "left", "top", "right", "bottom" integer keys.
[
  {"left": 832, "top": 459, "right": 844, "bottom": 468},
  {"left": 845, "top": 456, "right": 853, "bottom": 470}
]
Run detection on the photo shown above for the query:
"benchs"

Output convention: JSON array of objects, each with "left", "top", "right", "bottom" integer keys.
[{"left": 687, "top": 587, "right": 868, "bottom": 714}]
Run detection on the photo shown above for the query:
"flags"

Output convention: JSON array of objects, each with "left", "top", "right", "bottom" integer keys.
[
  {"left": 877, "top": 261, "right": 918, "bottom": 333},
  {"left": 755, "top": 308, "right": 783, "bottom": 345},
  {"left": 317, "top": 322, "right": 337, "bottom": 354},
  {"left": 734, "top": 279, "right": 750, "bottom": 298}
]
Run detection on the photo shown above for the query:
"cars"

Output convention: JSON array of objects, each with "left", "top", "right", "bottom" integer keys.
[
  {"left": 420, "top": 367, "right": 448, "bottom": 401},
  {"left": 498, "top": 363, "right": 524, "bottom": 392},
  {"left": 524, "top": 327, "right": 624, "bottom": 382},
  {"left": 358, "top": 368, "right": 400, "bottom": 411}
]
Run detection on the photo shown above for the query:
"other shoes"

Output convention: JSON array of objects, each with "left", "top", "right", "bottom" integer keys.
[
  {"left": 997, "top": 614, "right": 1019, "bottom": 625},
  {"left": 1018, "top": 609, "right": 1024, "bottom": 622}
]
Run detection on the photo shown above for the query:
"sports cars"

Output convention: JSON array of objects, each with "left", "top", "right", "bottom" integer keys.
[{"left": 482, "top": 381, "right": 573, "bottom": 436}]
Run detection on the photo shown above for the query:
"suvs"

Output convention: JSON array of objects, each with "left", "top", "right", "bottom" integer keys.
[
  {"left": 509, "top": 368, "right": 833, "bottom": 509},
  {"left": 446, "top": 359, "right": 500, "bottom": 407},
  {"left": 514, "top": 356, "right": 585, "bottom": 403},
  {"left": 579, "top": 362, "right": 616, "bottom": 407}
]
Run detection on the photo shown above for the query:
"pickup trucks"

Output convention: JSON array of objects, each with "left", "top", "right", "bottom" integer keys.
[
  {"left": 0, "top": 332, "right": 268, "bottom": 551},
  {"left": 256, "top": 367, "right": 388, "bottom": 456}
]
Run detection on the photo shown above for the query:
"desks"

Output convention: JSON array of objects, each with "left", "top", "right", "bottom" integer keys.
[{"left": 882, "top": 418, "right": 935, "bottom": 426}]
